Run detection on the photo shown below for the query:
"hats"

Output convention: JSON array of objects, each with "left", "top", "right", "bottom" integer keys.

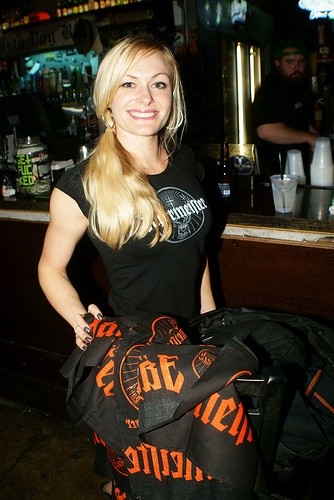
[{"left": 275, "top": 40, "right": 306, "bottom": 57}]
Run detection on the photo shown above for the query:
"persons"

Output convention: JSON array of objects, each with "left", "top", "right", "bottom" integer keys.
[
  {"left": 251, "top": 38, "right": 320, "bottom": 185},
  {"left": 38, "top": 37, "right": 217, "bottom": 496}
]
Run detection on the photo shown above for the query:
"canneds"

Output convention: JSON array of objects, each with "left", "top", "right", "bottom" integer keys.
[{"left": 16, "top": 143, "right": 51, "bottom": 197}]
